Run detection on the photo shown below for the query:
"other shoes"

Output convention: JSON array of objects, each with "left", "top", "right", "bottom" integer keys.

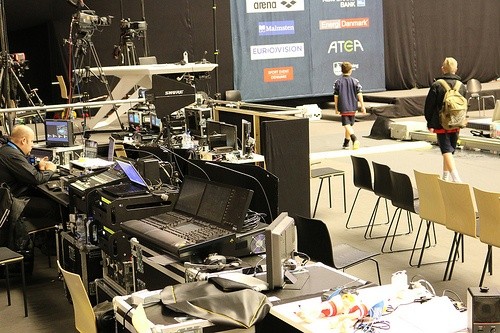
[
  {"left": 353, "top": 138, "right": 359, "bottom": 150},
  {"left": 343, "top": 144, "right": 350, "bottom": 149}
]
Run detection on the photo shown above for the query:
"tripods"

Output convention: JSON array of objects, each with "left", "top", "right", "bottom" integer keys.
[{"left": 65, "top": 26, "right": 124, "bottom": 132}]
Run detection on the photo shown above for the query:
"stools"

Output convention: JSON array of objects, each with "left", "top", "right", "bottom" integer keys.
[{"left": 0, "top": 246, "right": 28, "bottom": 317}]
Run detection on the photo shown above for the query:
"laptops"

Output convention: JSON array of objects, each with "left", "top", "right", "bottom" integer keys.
[{"left": 102, "top": 158, "right": 151, "bottom": 197}]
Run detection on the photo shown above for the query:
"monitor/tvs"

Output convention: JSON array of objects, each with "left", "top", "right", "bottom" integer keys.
[
  {"left": 264, "top": 211, "right": 310, "bottom": 290},
  {"left": 44, "top": 119, "right": 73, "bottom": 147},
  {"left": 173, "top": 175, "right": 254, "bottom": 233},
  {"left": 206, "top": 120, "right": 238, "bottom": 150},
  {"left": 242, "top": 119, "right": 253, "bottom": 159}
]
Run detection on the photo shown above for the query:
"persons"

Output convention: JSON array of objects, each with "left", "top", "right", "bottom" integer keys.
[
  {"left": 333, "top": 61, "right": 366, "bottom": 150},
  {"left": 424, "top": 57, "right": 467, "bottom": 183},
  {"left": 0, "top": 125, "right": 57, "bottom": 255}
]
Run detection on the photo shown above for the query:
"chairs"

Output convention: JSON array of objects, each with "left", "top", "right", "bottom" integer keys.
[
  {"left": 293, "top": 156, "right": 500, "bottom": 287},
  {"left": 56, "top": 259, "right": 115, "bottom": 333},
  {"left": 0, "top": 187, "right": 61, "bottom": 272}
]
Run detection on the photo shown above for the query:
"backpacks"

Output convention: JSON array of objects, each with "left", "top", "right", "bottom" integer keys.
[{"left": 437, "top": 79, "right": 468, "bottom": 129}]
